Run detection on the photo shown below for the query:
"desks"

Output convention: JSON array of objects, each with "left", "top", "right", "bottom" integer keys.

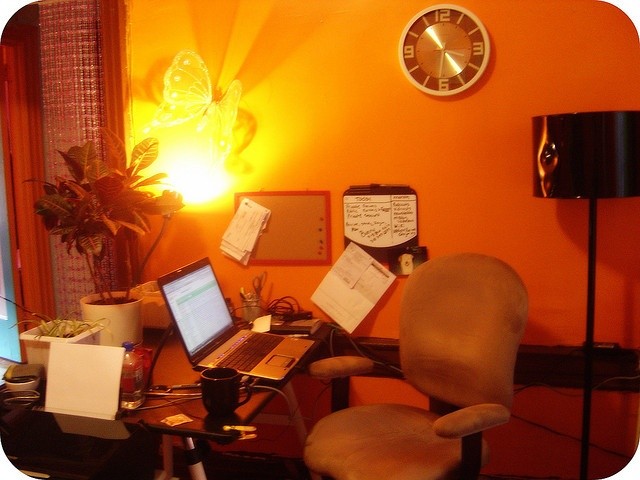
[{"left": 2, "top": 323, "right": 333, "bottom": 480}]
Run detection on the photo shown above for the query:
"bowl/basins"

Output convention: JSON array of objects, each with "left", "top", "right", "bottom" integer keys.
[{"left": 5, "top": 378, "right": 40, "bottom": 390}]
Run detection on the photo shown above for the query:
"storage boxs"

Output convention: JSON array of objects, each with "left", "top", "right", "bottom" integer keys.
[{"left": 129, "top": 280, "right": 173, "bottom": 329}]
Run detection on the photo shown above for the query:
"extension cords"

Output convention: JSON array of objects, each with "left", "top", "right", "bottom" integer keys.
[{"left": 282, "top": 311, "right": 313, "bottom": 322}]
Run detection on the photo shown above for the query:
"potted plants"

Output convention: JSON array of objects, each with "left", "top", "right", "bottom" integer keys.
[
  {"left": 23, "top": 137, "right": 185, "bottom": 349},
  {"left": 8, "top": 309, "right": 113, "bottom": 381}
]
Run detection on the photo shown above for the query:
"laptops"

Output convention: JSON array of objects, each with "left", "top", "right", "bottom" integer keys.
[{"left": 156, "top": 256, "right": 316, "bottom": 382}]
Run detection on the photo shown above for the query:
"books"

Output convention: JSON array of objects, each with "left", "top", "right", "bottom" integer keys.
[{"left": 271, "top": 318, "right": 322, "bottom": 335}]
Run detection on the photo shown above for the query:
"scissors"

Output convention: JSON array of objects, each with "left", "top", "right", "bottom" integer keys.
[{"left": 252, "top": 271, "right": 267, "bottom": 305}]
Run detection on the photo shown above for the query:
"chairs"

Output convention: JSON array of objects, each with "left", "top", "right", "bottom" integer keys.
[{"left": 302, "top": 253, "right": 530, "bottom": 479}]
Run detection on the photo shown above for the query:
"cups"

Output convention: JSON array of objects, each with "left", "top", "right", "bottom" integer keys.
[{"left": 200, "top": 367, "right": 252, "bottom": 417}]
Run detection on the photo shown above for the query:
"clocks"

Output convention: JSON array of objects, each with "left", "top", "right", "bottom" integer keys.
[{"left": 398, "top": 3, "right": 491, "bottom": 97}]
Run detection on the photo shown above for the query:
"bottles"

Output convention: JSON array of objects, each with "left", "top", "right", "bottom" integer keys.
[
  {"left": 226, "top": 298, "right": 236, "bottom": 317},
  {"left": 120, "top": 342, "right": 145, "bottom": 410}
]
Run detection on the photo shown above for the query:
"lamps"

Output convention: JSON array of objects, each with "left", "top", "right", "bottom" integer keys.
[{"left": 532, "top": 110, "right": 640, "bottom": 480}]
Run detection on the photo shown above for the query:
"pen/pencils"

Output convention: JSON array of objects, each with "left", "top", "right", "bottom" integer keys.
[
  {"left": 241, "top": 287, "right": 245, "bottom": 297},
  {"left": 239, "top": 292, "right": 247, "bottom": 301},
  {"left": 288, "top": 334, "right": 309, "bottom": 337},
  {"left": 173, "top": 384, "right": 202, "bottom": 388}
]
